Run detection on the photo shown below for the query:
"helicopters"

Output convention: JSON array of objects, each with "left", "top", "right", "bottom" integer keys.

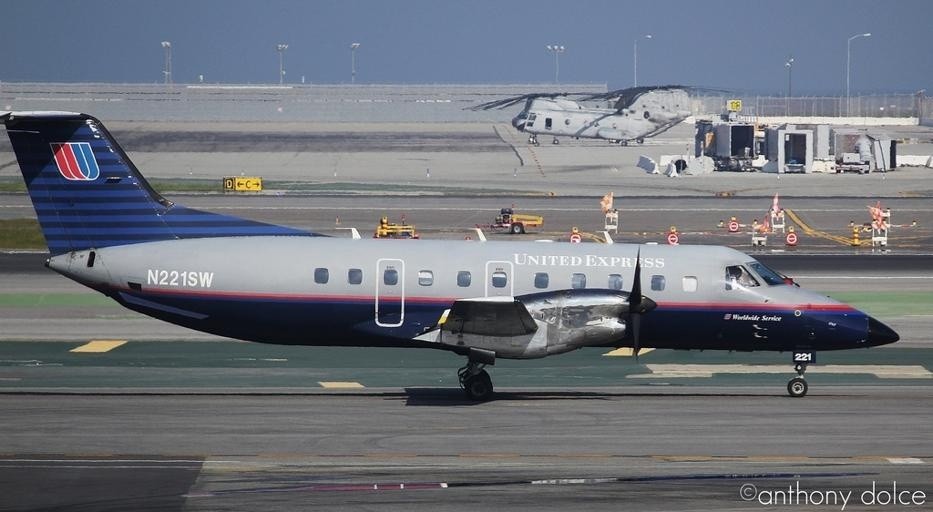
[{"left": 471, "top": 86, "right": 730, "bottom": 148}]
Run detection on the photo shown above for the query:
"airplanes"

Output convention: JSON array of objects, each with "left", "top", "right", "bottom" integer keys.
[{"left": 0, "top": 109, "right": 906, "bottom": 404}]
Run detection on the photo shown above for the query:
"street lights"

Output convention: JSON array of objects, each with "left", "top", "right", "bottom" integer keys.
[
  {"left": 159, "top": 40, "right": 175, "bottom": 84},
  {"left": 544, "top": 44, "right": 566, "bottom": 83},
  {"left": 632, "top": 33, "right": 652, "bottom": 88},
  {"left": 275, "top": 39, "right": 290, "bottom": 84},
  {"left": 349, "top": 42, "right": 362, "bottom": 82},
  {"left": 845, "top": 31, "right": 871, "bottom": 115},
  {"left": 782, "top": 56, "right": 795, "bottom": 97}
]
